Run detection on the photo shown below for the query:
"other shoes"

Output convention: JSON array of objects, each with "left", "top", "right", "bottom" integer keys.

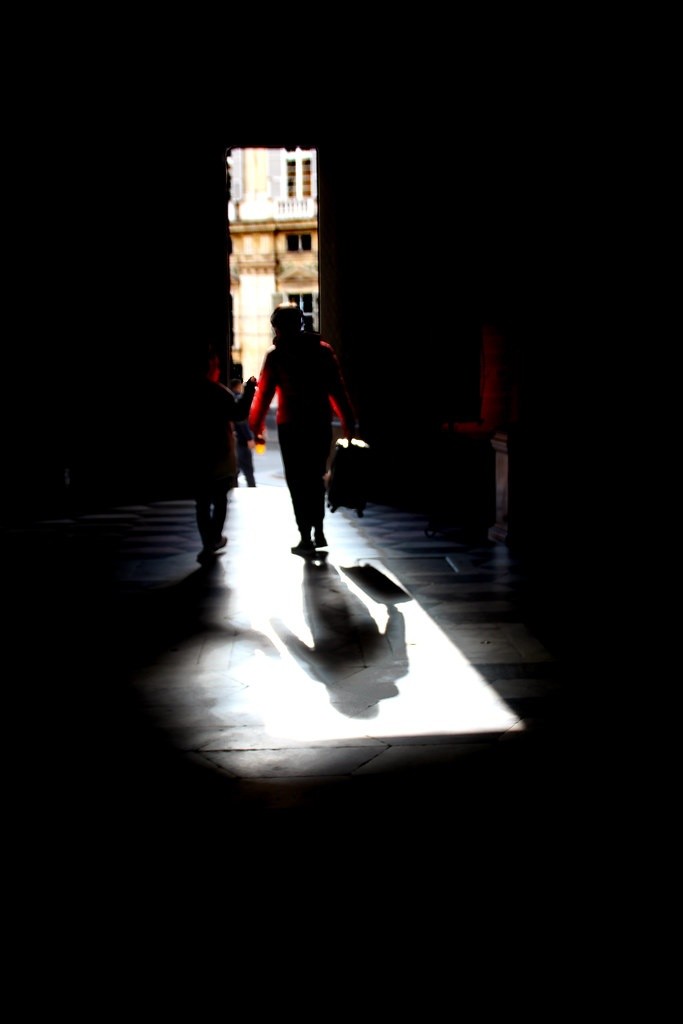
[
  {"left": 291, "top": 527, "right": 327, "bottom": 551},
  {"left": 198, "top": 535, "right": 227, "bottom": 565}
]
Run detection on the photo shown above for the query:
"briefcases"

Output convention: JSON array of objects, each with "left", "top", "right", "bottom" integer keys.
[{"left": 327, "top": 435, "right": 373, "bottom": 516}]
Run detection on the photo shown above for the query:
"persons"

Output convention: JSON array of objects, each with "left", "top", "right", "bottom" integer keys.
[{"left": 187, "top": 300, "right": 355, "bottom": 558}]
